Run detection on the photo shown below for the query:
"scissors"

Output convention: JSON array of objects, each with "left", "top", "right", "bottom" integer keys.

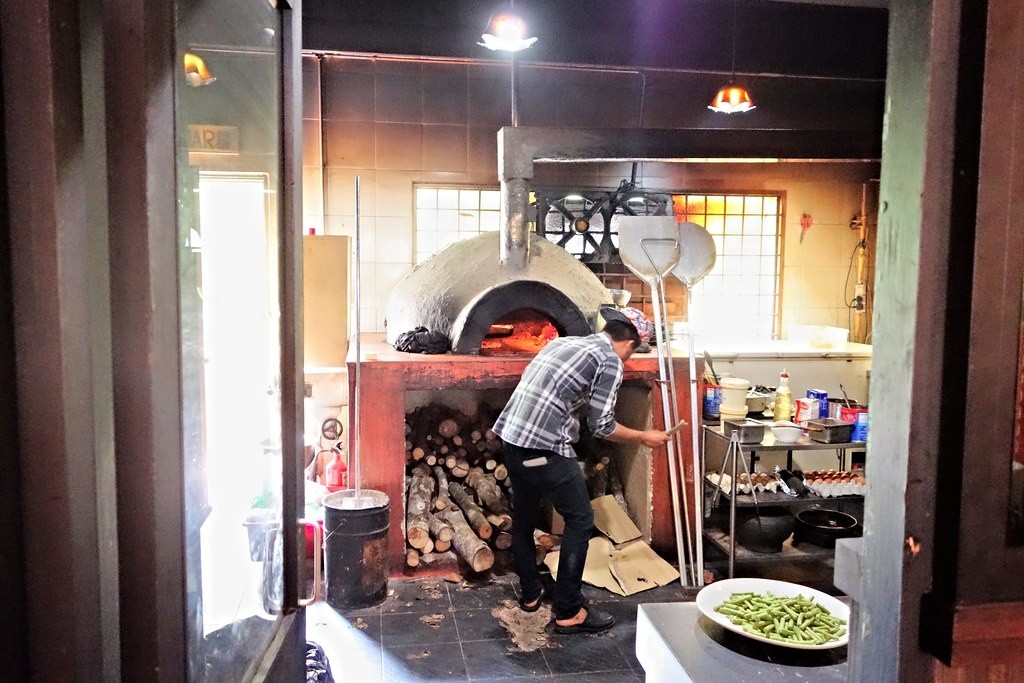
[{"left": 799, "top": 214, "right": 813, "bottom": 244}]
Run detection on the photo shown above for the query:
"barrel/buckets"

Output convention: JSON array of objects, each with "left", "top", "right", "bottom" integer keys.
[
  {"left": 703, "top": 376, "right": 771, "bottom": 444},
  {"left": 829, "top": 398, "right": 869, "bottom": 443},
  {"left": 808, "top": 417, "right": 851, "bottom": 445},
  {"left": 320, "top": 488, "right": 390, "bottom": 609}
]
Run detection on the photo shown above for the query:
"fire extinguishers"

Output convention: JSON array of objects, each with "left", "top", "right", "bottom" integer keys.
[{"left": 325, "top": 441, "right": 347, "bottom": 492}]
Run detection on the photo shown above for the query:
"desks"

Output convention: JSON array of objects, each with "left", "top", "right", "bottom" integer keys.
[{"left": 634, "top": 595, "right": 852, "bottom": 683}]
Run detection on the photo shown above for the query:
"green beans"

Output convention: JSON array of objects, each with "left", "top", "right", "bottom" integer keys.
[{"left": 713, "top": 591, "right": 846, "bottom": 645}]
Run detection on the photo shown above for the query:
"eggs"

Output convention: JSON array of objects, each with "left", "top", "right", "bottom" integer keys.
[{"left": 736, "top": 469, "right": 865, "bottom": 486}]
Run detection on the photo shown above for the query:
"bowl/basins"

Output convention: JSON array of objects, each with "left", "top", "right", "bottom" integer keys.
[
  {"left": 773, "top": 420, "right": 800, "bottom": 444},
  {"left": 798, "top": 506, "right": 856, "bottom": 548}
]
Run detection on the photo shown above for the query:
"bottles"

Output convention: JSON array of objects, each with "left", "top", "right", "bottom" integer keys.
[
  {"left": 775, "top": 369, "right": 792, "bottom": 421},
  {"left": 736, "top": 508, "right": 795, "bottom": 555}
]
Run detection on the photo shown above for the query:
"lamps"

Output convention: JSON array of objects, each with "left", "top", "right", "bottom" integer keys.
[
  {"left": 707, "top": 1, "right": 756, "bottom": 115},
  {"left": 477, "top": 1, "right": 540, "bottom": 52}
]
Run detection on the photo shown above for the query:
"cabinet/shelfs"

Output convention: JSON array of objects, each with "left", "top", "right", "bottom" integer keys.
[{"left": 700, "top": 416, "right": 867, "bottom": 582}]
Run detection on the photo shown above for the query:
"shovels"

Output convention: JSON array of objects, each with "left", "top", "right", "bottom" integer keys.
[
  {"left": 667, "top": 222, "right": 717, "bottom": 586},
  {"left": 704, "top": 351, "right": 719, "bottom": 385},
  {"left": 616, "top": 216, "right": 689, "bottom": 587}
]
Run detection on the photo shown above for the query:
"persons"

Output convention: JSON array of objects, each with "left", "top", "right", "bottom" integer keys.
[{"left": 493, "top": 307, "right": 671, "bottom": 633}]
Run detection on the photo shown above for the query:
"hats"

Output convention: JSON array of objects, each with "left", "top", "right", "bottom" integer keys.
[{"left": 599, "top": 306, "right": 655, "bottom": 345}]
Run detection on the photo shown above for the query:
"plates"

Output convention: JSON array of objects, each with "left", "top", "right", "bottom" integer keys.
[{"left": 696, "top": 573, "right": 851, "bottom": 652}]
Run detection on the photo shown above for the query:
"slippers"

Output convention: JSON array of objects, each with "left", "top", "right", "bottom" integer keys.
[
  {"left": 521, "top": 577, "right": 552, "bottom": 611},
  {"left": 553, "top": 605, "right": 616, "bottom": 633}
]
